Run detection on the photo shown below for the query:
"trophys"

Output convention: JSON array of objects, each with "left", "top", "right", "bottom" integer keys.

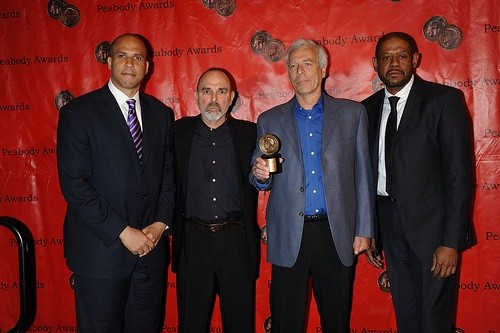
[{"left": 259, "top": 135, "right": 280, "bottom": 174}]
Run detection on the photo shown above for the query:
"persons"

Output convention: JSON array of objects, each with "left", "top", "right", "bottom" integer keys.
[
  {"left": 249, "top": 39, "right": 375, "bottom": 333},
  {"left": 57, "top": 32, "right": 176, "bottom": 333},
  {"left": 170, "top": 68, "right": 260, "bottom": 333},
  {"left": 360, "top": 32, "right": 476, "bottom": 333}
]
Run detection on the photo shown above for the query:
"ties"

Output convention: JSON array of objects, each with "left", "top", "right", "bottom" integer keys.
[
  {"left": 126, "top": 99, "right": 142, "bottom": 167},
  {"left": 384, "top": 96, "right": 400, "bottom": 201}
]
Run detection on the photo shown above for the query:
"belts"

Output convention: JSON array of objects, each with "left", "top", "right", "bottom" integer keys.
[
  {"left": 303, "top": 214, "right": 328, "bottom": 223},
  {"left": 186, "top": 215, "right": 243, "bottom": 232}
]
